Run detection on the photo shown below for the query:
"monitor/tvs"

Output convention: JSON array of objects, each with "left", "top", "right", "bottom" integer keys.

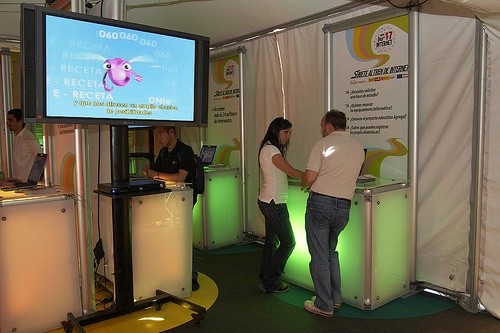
[{"left": 20, "top": 2, "right": 210, "bottom": 127}]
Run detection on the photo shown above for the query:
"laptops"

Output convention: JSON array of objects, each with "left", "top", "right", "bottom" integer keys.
[
  {"left": 2, "top": 153, "right": 48, "bottom": 191},
  {"left": 200, "top": 145, "right": 217, "bottom": 164}
]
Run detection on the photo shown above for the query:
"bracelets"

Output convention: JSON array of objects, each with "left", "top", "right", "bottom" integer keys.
[{"left": 158, "top": 172, "right": 159, "bottom": 179}]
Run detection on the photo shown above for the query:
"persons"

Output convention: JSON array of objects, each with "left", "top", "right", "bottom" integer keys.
[
  {"left": 302, "top": 109, "right": 365, "bottom": 317},
  {"left": 7, "top": 108, "right": 41, "bottom": 183},
  {"left": 143, "top": 125, "right": 200, "bottom": 292},
  {"left": 258, "top": 118, "right": 304, "bottom": 293}
]
